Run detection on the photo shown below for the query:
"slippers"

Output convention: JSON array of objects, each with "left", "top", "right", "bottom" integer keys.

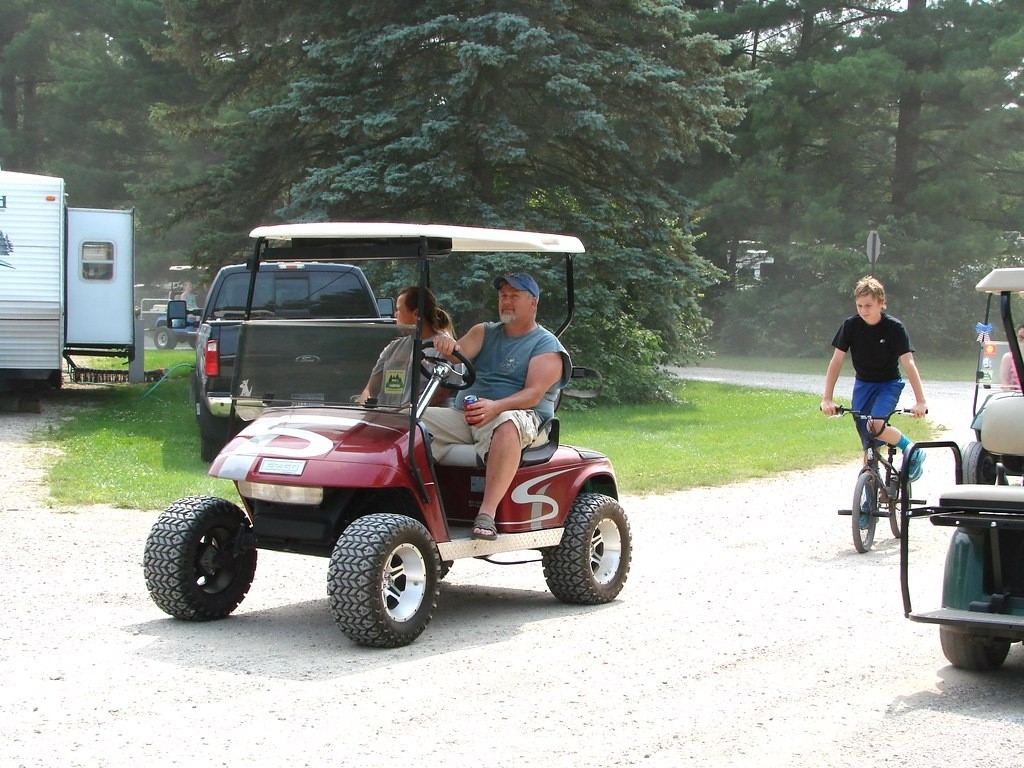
[{"left": 471, "top": 513, "right": 497, "bottom": 541}]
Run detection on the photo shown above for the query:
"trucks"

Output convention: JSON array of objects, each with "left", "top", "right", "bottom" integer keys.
[{"left": 0, "top": 169, "right": 135, "bottom": 390}]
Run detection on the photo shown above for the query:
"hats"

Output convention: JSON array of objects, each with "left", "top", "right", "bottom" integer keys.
[{"left": 494, "top": 272, "right": 540, "bottom": 304}]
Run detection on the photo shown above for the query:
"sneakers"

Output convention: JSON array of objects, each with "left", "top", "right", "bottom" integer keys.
[
  {"left": 859, "top": 509, "right": 879, "bottom": 530},
  {"left": 908, "top": 448, "right": 928, "bottom": 483}
]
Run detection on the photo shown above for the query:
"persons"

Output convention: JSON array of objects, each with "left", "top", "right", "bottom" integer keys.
[
  {"left": 182, "top": 278, "right": 202, "bottom": 323},
  {"left": 424, "top": 271, "right": 573, "bottom": 542},
  {"left": 357, "top": 284, "right": 461, "bottom": 413},
  {"left": 999, "top": 324, "right": 1024, "bottom": 392},
  {"left": 820, "top": 275, "right": 928, "bottom": 534}
]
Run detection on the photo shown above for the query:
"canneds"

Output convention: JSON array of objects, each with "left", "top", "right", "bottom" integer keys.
[{"left": 464, "top": 395, "right": 480, "bottom": 424}]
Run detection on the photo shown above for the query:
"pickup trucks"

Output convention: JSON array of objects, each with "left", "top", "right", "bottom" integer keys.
[{"left": 166, "top": 260, "right": 417, "bottom": 463}]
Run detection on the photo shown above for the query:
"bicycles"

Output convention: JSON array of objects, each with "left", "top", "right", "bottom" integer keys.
[{"left": 819, "top": 405, "right": 928, "bottom": 553}]
